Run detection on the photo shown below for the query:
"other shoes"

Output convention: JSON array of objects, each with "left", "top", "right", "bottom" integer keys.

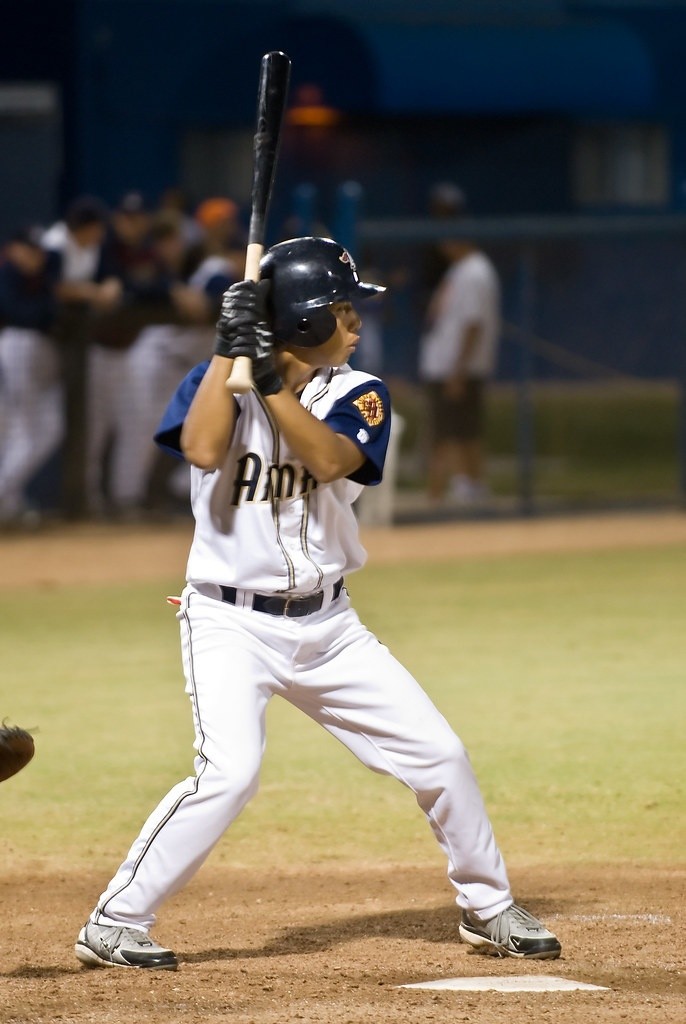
[{"left": 447, "top": 477, "right": 493, "bottom": 510}]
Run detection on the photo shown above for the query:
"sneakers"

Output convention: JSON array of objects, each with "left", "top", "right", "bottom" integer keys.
[
  {"left": 459, "top": 901, "right": 561, "bottom": 959},
  {"left": 74, "top": 917, "right": 178, "bottom": 972}
]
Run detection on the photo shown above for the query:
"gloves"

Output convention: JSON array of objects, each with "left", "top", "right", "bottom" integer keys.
[
  {"left": 223, "top": 313, "right": 285, "bottom": 397},
  {"left": 216, "top": 279, "right": 271, "bottom": 359}
]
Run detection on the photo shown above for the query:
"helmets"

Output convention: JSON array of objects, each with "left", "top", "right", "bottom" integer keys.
[{"left": 259, "top": 236, "right": 388, "bottom": 347}]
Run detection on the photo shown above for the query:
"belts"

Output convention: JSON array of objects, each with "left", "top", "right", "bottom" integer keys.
[{"left": 220, "top": 577, "right": 344, "bottom": 616}]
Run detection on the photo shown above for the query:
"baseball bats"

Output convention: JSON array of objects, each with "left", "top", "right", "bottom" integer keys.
[{"left": 221, "top": 50, "right": 294, "bottom": 395}]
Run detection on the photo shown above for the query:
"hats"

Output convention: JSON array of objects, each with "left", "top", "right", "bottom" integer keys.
[{"left": 113, "top": 183, "right": 162, "bottom": 216}]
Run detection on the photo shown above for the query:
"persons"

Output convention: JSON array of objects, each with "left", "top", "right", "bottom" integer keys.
[
  {"left": 0, "top": 193, "right": 500, "bottom": 533},
  {"left": 71, "top": 235, "right": 561, "bottom": 970}
]
